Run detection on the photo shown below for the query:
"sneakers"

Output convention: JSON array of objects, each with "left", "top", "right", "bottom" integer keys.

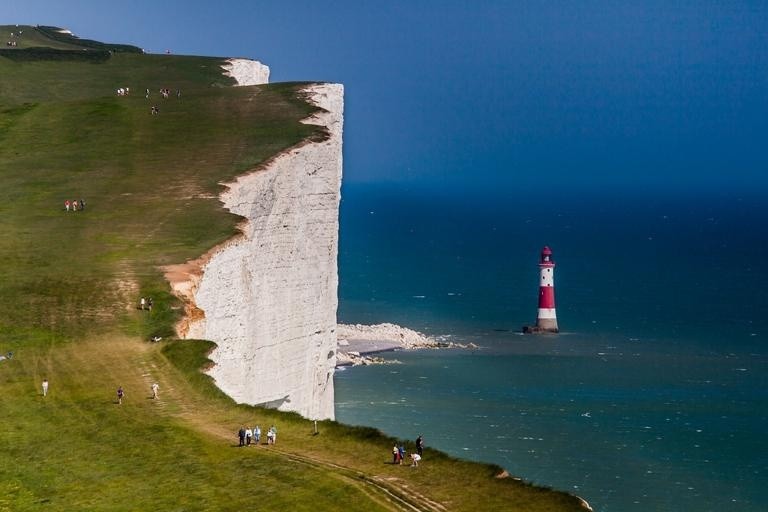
[{"left": 411, "top": 464, "right": 418, "bottom": 466}]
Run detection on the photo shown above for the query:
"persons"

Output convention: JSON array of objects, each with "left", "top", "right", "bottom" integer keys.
[
  {"left": 392, "top": 443, "right": 399, "bottom": 463},
  {"left": 64, "top": 199, "right": 71, "bottom": 212},
  {"left": 398, "top": 444, "right": 406, "bottom": 464},
  {"left": 151, "top": 382, "right": 160, "bottom": 399},
  {"left": 151, "top": 335, "right": 162, "bottom": 342},
  {"left": 72, "top": 200, "right": 78, "bottom": 211},
  {"left": 115, "top": 86, "right": 181, "bottom": 115},
  {"left": 79, "top": 199, "right": 85, "bottom": 210},
  {"left": 7, "top": 24, "right": 25, "bottom": 48},
  {"left": 408, "top": 453, "right": 421, "bottom": 467},
  {"left": 42, "top": 379, "right": 48, "bottom": 396},
  {"left": 117, "top": 385, "right": 124, "bottom": 406},
  {"left": 0, "top": 351, "right": 13, "bottom": 363},
  {"left": 239, "top": 423, "right": 279, "bottom": 447},
  {"left": 147, "top": 297, "right": 154, "bottom": 311},
  {"left": 416, "top": 436, "right": 424, "bottom": 455},
  {"left": 140, "top": 296, "right": 146, "bottom": 309}
]
